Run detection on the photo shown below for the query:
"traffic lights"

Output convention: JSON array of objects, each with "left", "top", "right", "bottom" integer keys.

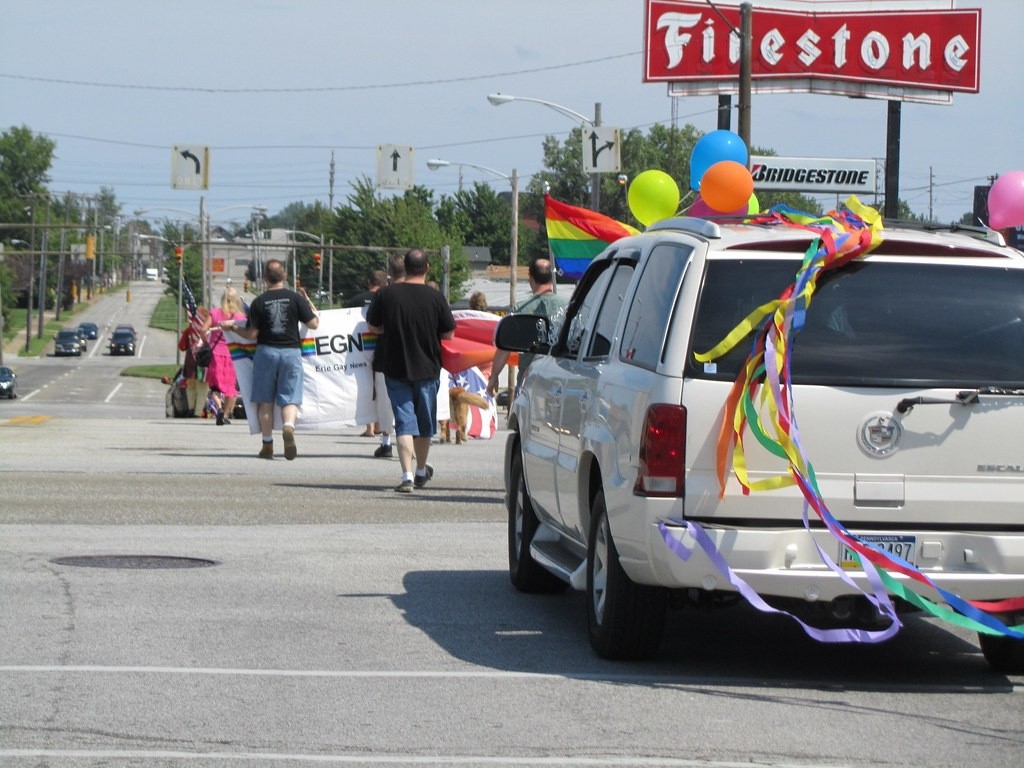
[
  {"left": 175, "top": 247, "right": 183, "bottom": 266},
  {"left": 295, "top": 279, "right": 301, "bottom": 291},
  {"left": 314, "top": 253, "right": 321, "bottom": 272},
  {"left": 244, "top": 281, "right": 248, "bottom": 292}
]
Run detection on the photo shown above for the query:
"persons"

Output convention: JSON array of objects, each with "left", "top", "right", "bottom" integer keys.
[
  {"left": 219, "top": 259, "right": 319, "bottom": 460},
  {"left": 470, "top": 292, "right": 494, "bottom": 377},
  {"left": 365, "top": 248, "right": 457, "bottom": 492},
  {"left": 348, "top": 254, "right": 440, "bottom": 457},
  {"left": 179, "top": 308, "right": 210, "bottom": 417},
  {"left": 199, "top": 288, "right": 247, "bottom": 424},
  {"left": 486, "top": 258, "right": 569, "bottom": 401}
]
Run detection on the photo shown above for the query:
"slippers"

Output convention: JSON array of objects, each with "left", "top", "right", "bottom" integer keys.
[{"left": 361, "top": 431, "right": 374, "bottom": 438}]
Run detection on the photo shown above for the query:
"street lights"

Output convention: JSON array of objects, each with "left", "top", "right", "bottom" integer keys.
[
  {"left": 487, "top": 93, "right": 603, "bottom": 217},
  {"left": 9, "top": 238, "right": 35, "bottom": 353},
  {"left": 422, "top": 160, "right": 520, "bottom": 423},
  {"left": 130, "top": 205, "right": 269, "bottom": 310}
]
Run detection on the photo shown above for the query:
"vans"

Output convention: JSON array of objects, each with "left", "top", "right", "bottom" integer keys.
[
  {"left": 145, "top": 266, "right": 159, "bottom": 282},
  {"left": 52, "top": 328, "right": 82, "bottom": 356}
]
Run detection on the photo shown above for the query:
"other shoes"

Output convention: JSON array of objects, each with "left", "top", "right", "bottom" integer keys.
[
  {"left": 215, "top": 409, "right": 224, "bottom": 426},
  {"left": 259, "top": 439, "right": 274, "bottom": 459},
  {"left": 282, "top": 427, "right": 296, "bottom": 460},
  {"left": 224, "top": 417, "right": 231, "bottom": 425}
]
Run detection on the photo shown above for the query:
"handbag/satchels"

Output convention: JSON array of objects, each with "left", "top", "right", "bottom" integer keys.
[{"left": 196, "top": 344, "right": 212, "bottom": 369}]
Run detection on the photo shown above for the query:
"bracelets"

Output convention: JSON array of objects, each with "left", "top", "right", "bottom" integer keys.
[{"left": 230, "top": 325, "right": 234, "bottom": 331}]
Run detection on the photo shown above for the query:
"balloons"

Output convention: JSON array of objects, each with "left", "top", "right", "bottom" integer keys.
[
  {"left": 627, "top": 169, "right": 679, "bottom": 226},
  {"left": 689, "top": 129, "right": 748, "bottom": 190},
  {"left": 688, "top": 191, "right": 759, "bottom": 223},
  {"left": 700, "top": 160, "right": 754, "bottom": 212},
  {"left": 987, "top": 171, "right": 1024, "bottom": 229}
]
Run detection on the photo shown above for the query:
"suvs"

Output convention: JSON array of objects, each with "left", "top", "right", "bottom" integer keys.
[{"left": 494, "top": 214, "right": 1023, "bottom": 676}]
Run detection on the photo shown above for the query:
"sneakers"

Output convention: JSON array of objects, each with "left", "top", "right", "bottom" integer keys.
[
  {"left": 414, "top": 464, "right": 434, "bottom": 486},
  {"left": 395, "top": 480, "right": 414, "bottom": 492},
  {"left": 374, "top": 444, "right": 393, "bottom": 457}
]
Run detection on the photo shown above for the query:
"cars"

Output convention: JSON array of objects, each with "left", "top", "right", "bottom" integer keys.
[
  {"left": 161, "top": 365, "right": 246, "bottom": 420},
  {"left": 80, "top": 322, "right": 98, "bottom": 339},
  {"left": 77, "top": 327, "right": 89, "bottom": 352},
  {"left": 108, "top": 329, "right": 136, "bottom": 356},
  {"left": 0, "top": 367, "right": 18, "bottom": 399},
  {"left": 115, "top": 323, "right": 138, "bottom": 337}
]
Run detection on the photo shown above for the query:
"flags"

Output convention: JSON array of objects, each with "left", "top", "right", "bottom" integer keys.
[
  {"left": 183, "top": 283, "right": 207, "bottom": 360},
  {"left": 544, "top": 195, "right": 641, "bottom": 279},
  {"left": 448, "top": 364, "right": 498, "bottom": 439}
]
